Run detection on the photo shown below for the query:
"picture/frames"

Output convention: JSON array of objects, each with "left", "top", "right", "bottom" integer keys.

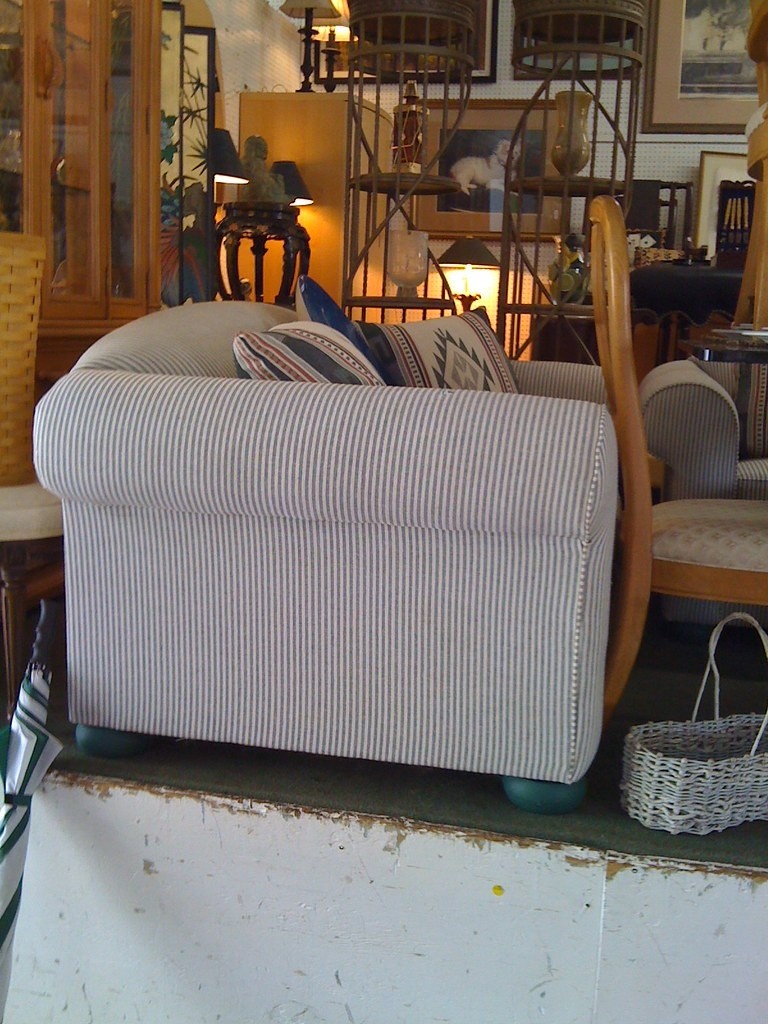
[
  {"left": 407, "top": 96, "right": 572, "bottom": 241},
  {"left": 690, "top": 149, "right": 755, "bottom": 262},
  {"left": 640, "top": 0, "right": 761, "bottom": 135}
]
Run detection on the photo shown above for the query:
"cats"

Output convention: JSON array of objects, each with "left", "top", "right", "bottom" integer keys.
[{"left": 450, "top": 138, "right": 520, "bottom": 195}]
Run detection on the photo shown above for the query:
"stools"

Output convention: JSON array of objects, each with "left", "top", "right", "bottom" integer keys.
[{"left": 208, "top": 202, "right": 310, "bottom": 311}]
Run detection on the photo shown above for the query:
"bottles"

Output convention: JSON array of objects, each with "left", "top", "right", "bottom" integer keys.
[
  {"left": 551, "top": 90, "right": 593, "bottom": 176},
  {"left": 391, "top": 79, "right": 430, "bottom": 175},
  {"left": 685, "top": 237, "right": 697, "bottom": 266},
  {"left": 550, "top": 233, "right": 588, "bottom": 305},
  {"left": 627, "top": 235, "right": 680, "bottom": 269}
]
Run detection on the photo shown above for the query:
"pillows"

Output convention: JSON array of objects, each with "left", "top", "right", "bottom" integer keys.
[
  {"left": 359, "top": 303, "right": 522, "bottom": 396},
  {"left": 232, "top": 317, "right": 384, "bottom": 387}
]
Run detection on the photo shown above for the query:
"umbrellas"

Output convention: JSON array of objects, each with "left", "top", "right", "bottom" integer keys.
[{"left": 0, "top": 598, "right": 68, "bottom": 1024}]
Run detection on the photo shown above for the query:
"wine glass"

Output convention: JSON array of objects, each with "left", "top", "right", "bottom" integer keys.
[{"left": 388, "top": 229, "right": 428, "bottom": 298}]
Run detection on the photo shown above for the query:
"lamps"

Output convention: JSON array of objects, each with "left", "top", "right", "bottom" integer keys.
[
  {"left": 431, "top": 239, "right": 501, "bottom": 269},
  {"left": 270, "top": 160, "right": 314, "bottom": 207},
  {"left": 309, "top": 0, "right": 350, "bottom": 93},
  {"left": 280, "top": 0, "right": 344, "bottom": 91}
]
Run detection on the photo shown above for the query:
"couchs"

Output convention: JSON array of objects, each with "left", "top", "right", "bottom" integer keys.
[
  {"left": 640, "top": 338, "right": 768, "bottom": 632},
  {"left": 31, "top": 293, "right": 628, "bottom": 809}
]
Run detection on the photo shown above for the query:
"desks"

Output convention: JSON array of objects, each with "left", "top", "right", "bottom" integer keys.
[{"left": 675, "top": 336, "right": 768, "bottom": 459}]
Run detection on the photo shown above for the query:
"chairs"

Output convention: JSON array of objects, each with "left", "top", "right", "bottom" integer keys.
[
  {"left": 0, "top": 230, "right": 64, "bottom": 730},
  {"left": 587, "top": 194, "right": 768, "bottom": 735}
]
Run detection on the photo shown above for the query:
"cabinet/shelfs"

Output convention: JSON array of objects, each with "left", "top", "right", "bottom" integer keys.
[
  {"left": 0, "top": 0, "right": 168, "bottom": 395},
  {"left": 239, "top": 91, "right": 392, "bottom": 325}
]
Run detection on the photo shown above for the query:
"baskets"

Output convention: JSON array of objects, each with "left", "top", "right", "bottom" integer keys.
[
  {"left": 0, "top": 231, "right": 44, "bottom": 486},
  {"left": 618, "top": 611, "right": 768, "bottom": 835}
]
